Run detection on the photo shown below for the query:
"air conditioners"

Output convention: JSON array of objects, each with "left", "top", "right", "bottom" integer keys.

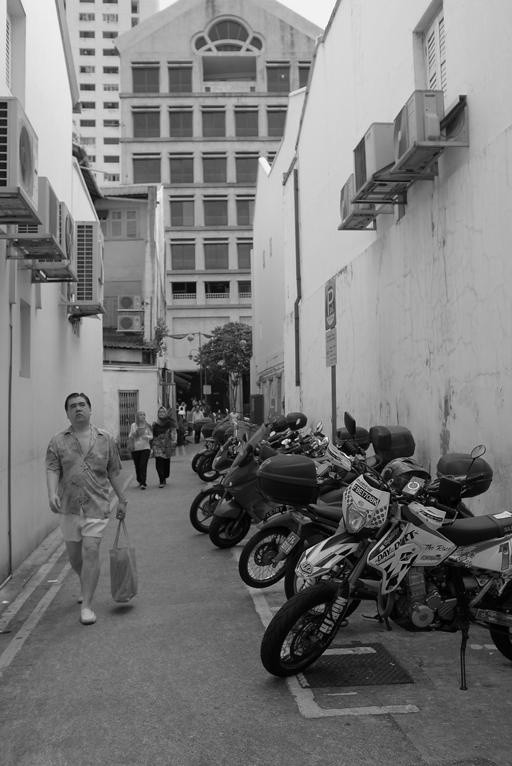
[
  {"left": 36, "top": 201, "right": 77, "bottom": 280},
  {"left": 339, "top": 173, "right": 375, "bottom": 228},
  {"left": 165, "top": 369, "right": 174, "bottom": 383},
  {"left": 393, "top": 89, "right": 447, "bottom": 171},
  {"left": 6, "top": 176, "right": 59, "bottom": 256},
  {"left": 69, "top": 220, "right": 105, "bottom": 312},
  {"left": 0, "top": 96, "right": 39, "bottom": 219},
  {"left": 117, "top": 315, "right": 141, "bottom": 330},
  {"left": 352, "top": 122, "right": 407, "bottom": 200},
  {"left": 117, "top": 295, "right": 141, "bottom": 309}
]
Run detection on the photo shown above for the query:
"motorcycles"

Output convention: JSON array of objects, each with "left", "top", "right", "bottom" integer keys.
[
  {"left": 189, "top": 413, "right": 493, "bottom": 618},
  {"left": 260, "top": 413, "right": 512, "bottom": 691}
]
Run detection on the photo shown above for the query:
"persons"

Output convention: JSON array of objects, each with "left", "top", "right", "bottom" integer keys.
[
  {"left": 151, "top": 407, "right": 177, "bottom": 488},
  {"left": 128, "top": 411, "right": 153, "bottom": 490},
  {"left": 45, "top": 395, "right": 127, "bottom": 624},
  {"left": 176, "top": 396, "right": 239, "bottom": 446}
]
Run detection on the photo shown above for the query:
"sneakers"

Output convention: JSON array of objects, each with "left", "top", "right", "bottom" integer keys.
[{"left": 79, "top": 595, "right": 96, "bottom": 624}]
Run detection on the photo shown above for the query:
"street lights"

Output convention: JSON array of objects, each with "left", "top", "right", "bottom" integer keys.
[
  {"left": 189, "top": 348, "right": 208, "bottom": 404},
  {"left": 187, "top": 331, "right": 215, "bottom": 402}
]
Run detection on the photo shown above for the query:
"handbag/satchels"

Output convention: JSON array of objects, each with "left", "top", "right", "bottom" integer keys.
[{"left": 109, "top": 547, "right": 138, "bottom": 603}]
